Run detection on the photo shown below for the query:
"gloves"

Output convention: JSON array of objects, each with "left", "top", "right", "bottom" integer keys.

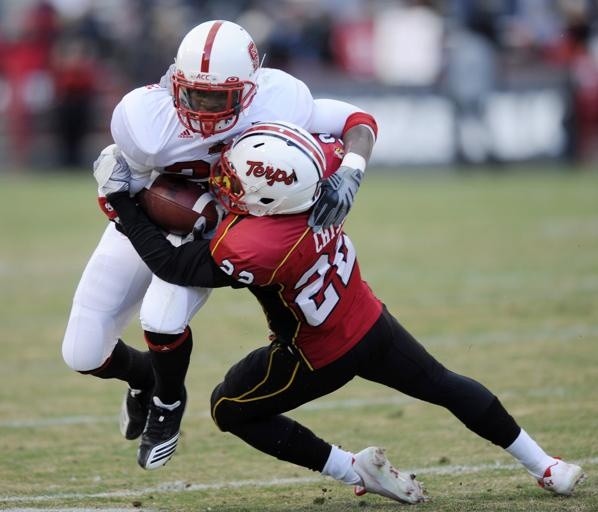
[
  {"left": 307, "top": 164, "right": 365, "bottom": 233},
  {"left": 91, "top": 143, "right": 131, "bottom": 195}
]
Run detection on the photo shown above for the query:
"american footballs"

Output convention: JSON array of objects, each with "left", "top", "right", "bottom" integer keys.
[{"left": 134, "top": 174, "right": 218, "bottom": 238}]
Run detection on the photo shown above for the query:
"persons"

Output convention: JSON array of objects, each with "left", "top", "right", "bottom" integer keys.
[
  {"left": 63, "top": 2, "right": 585, "bottom": 170},
  {"left": 97, "top": 122, "right": 585, "bottom": 508},
  {"left": 62, "top": 21, "right": 377, "bottom": 470}
]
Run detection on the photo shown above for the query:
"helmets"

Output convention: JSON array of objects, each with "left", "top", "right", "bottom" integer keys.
[
  {"left": 224, "top": 121, "right": 327, "bottom": 217},
  {"left": 170, "top": 19, "right": 259, "bottom": 94}
]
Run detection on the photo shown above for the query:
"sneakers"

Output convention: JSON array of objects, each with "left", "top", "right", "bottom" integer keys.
[
  {"left": 537, "top": 456, "right": 585, "bottom": 498},
  {"left": 119, "top": 374, "right": 187, "bottom": 470},
  {"left": 351, "top": 447, "right": 429, "bottom": 505}
]
